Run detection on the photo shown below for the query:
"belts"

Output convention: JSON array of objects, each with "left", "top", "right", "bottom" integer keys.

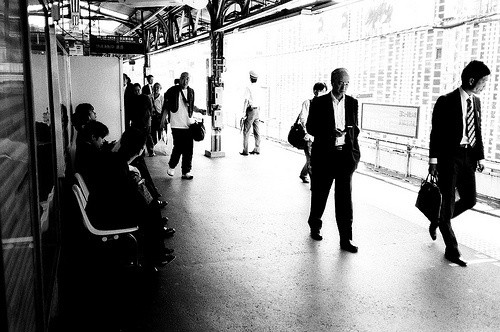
[
  {"left": 336, "top": 145, "right": 350, "bottom": 150},
  {"left": 461, "top": 144, "right": 472, "bottom": 148}
]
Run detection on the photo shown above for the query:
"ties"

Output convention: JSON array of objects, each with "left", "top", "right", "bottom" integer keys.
[{"left": 466, "top": 99, "right": 477, "bottom": 148}]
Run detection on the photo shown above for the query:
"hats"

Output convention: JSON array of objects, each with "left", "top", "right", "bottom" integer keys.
[{"left": 249, "top": 70, "right": 258, "bottom": 79}]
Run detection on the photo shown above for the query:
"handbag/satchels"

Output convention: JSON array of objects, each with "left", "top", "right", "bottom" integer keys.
[
  {"left": 192, "top": 122, "right": 206, "bottom": 141},
  {"left": 415, "top": 173, "right": 443, "bottom": 223},
  {"left": 287, "top": 123, "right": 307, "bottom": 149}
]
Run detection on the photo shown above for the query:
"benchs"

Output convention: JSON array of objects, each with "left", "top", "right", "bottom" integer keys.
[{"left": 73, "top": 173, "right": 141, "bottom": 273}]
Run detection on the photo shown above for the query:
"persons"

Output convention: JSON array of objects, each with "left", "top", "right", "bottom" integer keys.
[
  {"left": 299, "top": 82, "right": 328, "bottom": 191},
  {"left": 159, "top": 71, "right": 207, "bottom": 180},
  {"left": 305, "top": 67, "right": 361, "bottom": 253},
  {"left": 238, "top": 71, "right": 262, "bottom": 156},
  {"left": 429, "top": 59, "right": 490, "bottom": 267},
  {"left": 72, "top": 102, "right": 176, "bottom": 272},
  {"left": 123, "top": 71, "right": 164, "bottom": 157}
]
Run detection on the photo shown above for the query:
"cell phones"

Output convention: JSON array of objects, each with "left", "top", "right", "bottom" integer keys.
[{"left": 341, "top": 126, "right": 353, "bottom": 133}]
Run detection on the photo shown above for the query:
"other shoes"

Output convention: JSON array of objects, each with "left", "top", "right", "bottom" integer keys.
[
  {"left": 181, "top": 172, "right": 193, "bottom": 179},
  {"left": 429, "top": 223, "right": 437, "bottom": 241},
  {"left": 300, "top": 176, "right": 309, "bottom": 183},
  {"left": 311, "top": 229, "right": 322, "bottom": 241},
  {"left": 249, "top": 150, "right": 260, "bottom": 155},
  {"left": 239, "top": 151, "right": 248, "bottom": 156},
  {"left": 167, "top": 166, "right": 175, "bottom": 176},
  {"left": 445, "top": 254, "right": 468, "bottom": 267},
  {"left": 157, "top": 200, "right": 177, "bottom": 266},
  {"left": 148, "top": 152, "right": 155, "bottom": 157},
  {"left": 340, "top": 241, "right": 358, "bottom": 253}
]
